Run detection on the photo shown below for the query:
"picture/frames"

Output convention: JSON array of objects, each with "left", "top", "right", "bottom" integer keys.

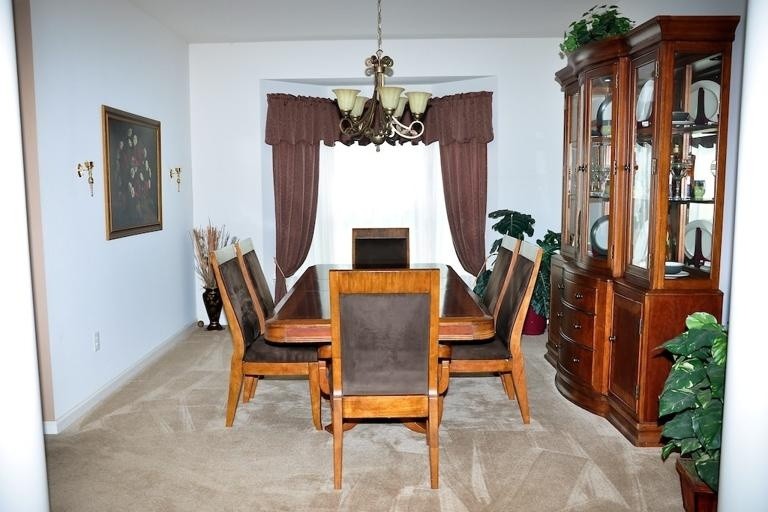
[{"left": 100, "top": 103, "right": 164, "bottom": 242}]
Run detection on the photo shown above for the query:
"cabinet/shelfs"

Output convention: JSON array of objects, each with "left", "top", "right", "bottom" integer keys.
[{"left": 542, "top": 14, "right": 743, "bottom": 449}]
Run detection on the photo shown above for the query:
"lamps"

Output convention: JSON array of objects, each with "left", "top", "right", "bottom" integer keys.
[
  {"left": 169, "top": 163, "right": 185, "bottom": 194},
  {"left": 74, "top": 160, "right": 98, "bottom": 198},
  {"left": 330, "top": 0, "right": 433, "bottom": 154}
]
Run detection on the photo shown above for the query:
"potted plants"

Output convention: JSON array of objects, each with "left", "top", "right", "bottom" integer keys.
[
  {"left": 472, "top": 208, "right": 561, "bottom": 336},
  {"left": 650, "top": 311, "right": 729, "bottom": 512},
  {"left": 188, "top": 219, "right": 239, "bottom": 333}
]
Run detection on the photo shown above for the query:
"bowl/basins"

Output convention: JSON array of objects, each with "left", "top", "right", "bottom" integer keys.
[
  {"left": 671, "top": 110, "right": 690, "bottom": 120},
  {"left": 665, "top": 262, "right": 684, "bottom": 274}
]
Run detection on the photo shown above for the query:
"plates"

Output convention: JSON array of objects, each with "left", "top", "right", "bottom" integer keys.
[
  {"left": 590, "top": 215, "right": 609, "bottom": 256},
  {"left": 596, "top": 95, "right": 613, "bottom": 135},
  {"left": 672, "top": 120, "right": 694, "bottom": 125},
  {"left": 684, "top": 220, "right": 713, "bottom": 261},
  {"left": 680, "top": 80, "right": 720, "bottom": 122},
  {"left": 636, "top": 80, "right": 654, "bottom": 122},
  {"left": 663, "top": 272, "right": 689, "bottom": 280}
]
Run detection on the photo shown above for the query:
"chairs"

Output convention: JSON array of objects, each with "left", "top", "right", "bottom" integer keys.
[
  {"left": 231, "top": 236, "right": 275, "bottom": 399},
  {"left": 479, "top": 234, "right": 521, "bottom": 332},
  {"left": 326, "top": 269, "right": 442, "bottom": 490},
  {"left": 438, "top": 238, "right": 544, "bottom": 426},
  {"left": 206, "top": 245, "right": 328, "bottom": 432},
  {"left": 351, "top": 227, "right": 410, "bottom": 264}
]
